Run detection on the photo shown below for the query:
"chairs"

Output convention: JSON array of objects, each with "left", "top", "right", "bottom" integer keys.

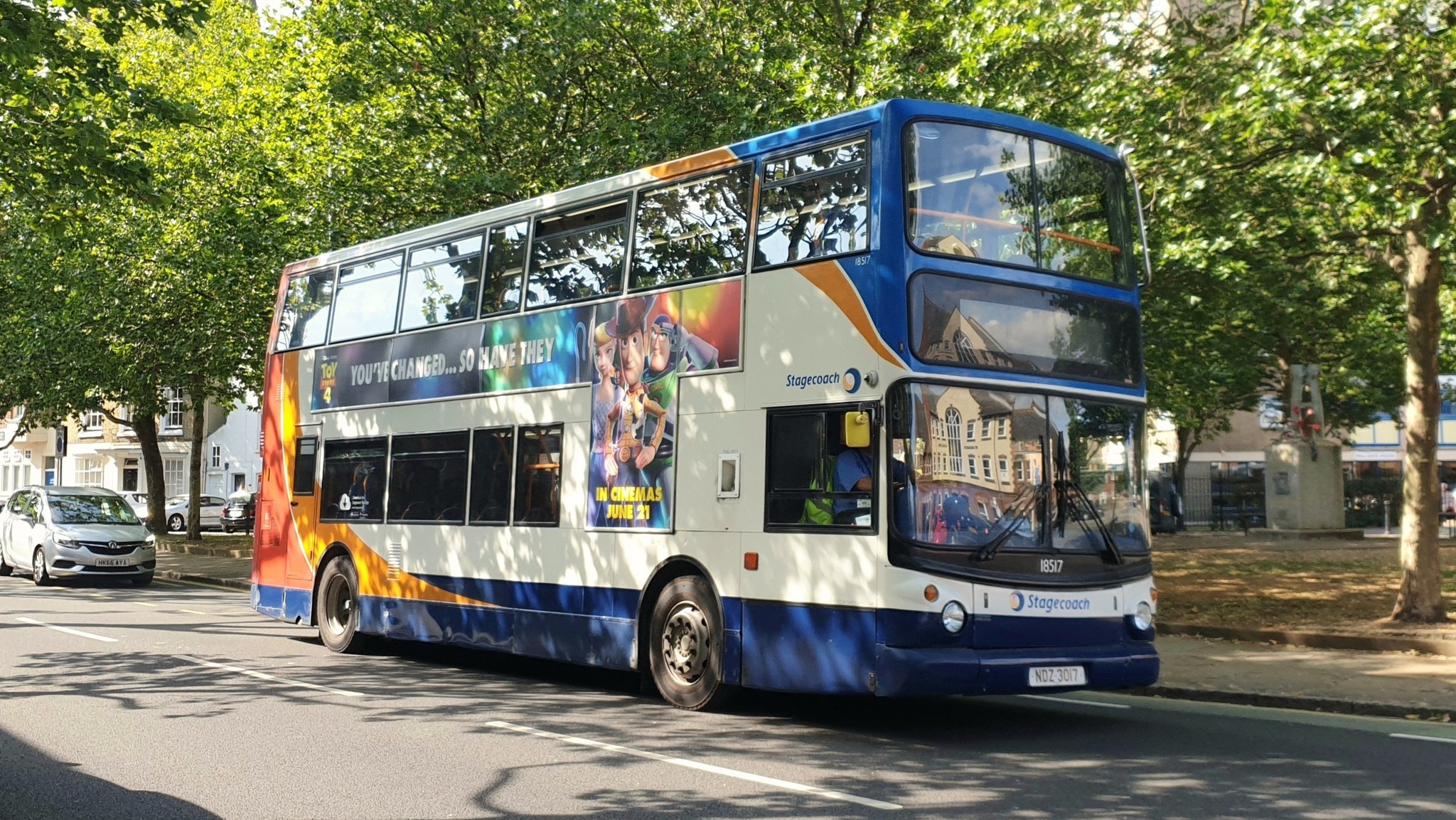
[{"left": 328, "top": 462, "right": 558, "bottom": 522}]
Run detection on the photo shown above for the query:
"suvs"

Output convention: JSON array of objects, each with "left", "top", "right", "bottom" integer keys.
[{"left": 1147, "top": 469, "right": 1183, "bottom": 535}]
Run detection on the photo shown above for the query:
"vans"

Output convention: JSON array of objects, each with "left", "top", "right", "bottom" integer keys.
[{"left": 220, "top": 490, "right": 257, "bottom": 534}]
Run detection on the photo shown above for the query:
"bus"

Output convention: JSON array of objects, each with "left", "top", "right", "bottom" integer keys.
[{"left": 248, "top": 97, "right": 1163, "bottom": 711}]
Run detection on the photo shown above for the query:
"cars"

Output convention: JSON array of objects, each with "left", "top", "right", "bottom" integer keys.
[
  {"left": 164, "top": 494, "right": 227, "bottom": 532},
  {"left": 0, "top": 484, "right": 158, "bottom": 586},
  {"left": 101, "top": 491, "right": 169, "bottom": 522}
]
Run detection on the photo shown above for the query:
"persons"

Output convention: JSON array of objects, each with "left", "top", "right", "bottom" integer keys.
[
  {"left": 1441, "top": 483, "right": 1454, "bottom": 512},
  {"left": 830, "top": 444, "right": 921, "bottom": 524}
]
